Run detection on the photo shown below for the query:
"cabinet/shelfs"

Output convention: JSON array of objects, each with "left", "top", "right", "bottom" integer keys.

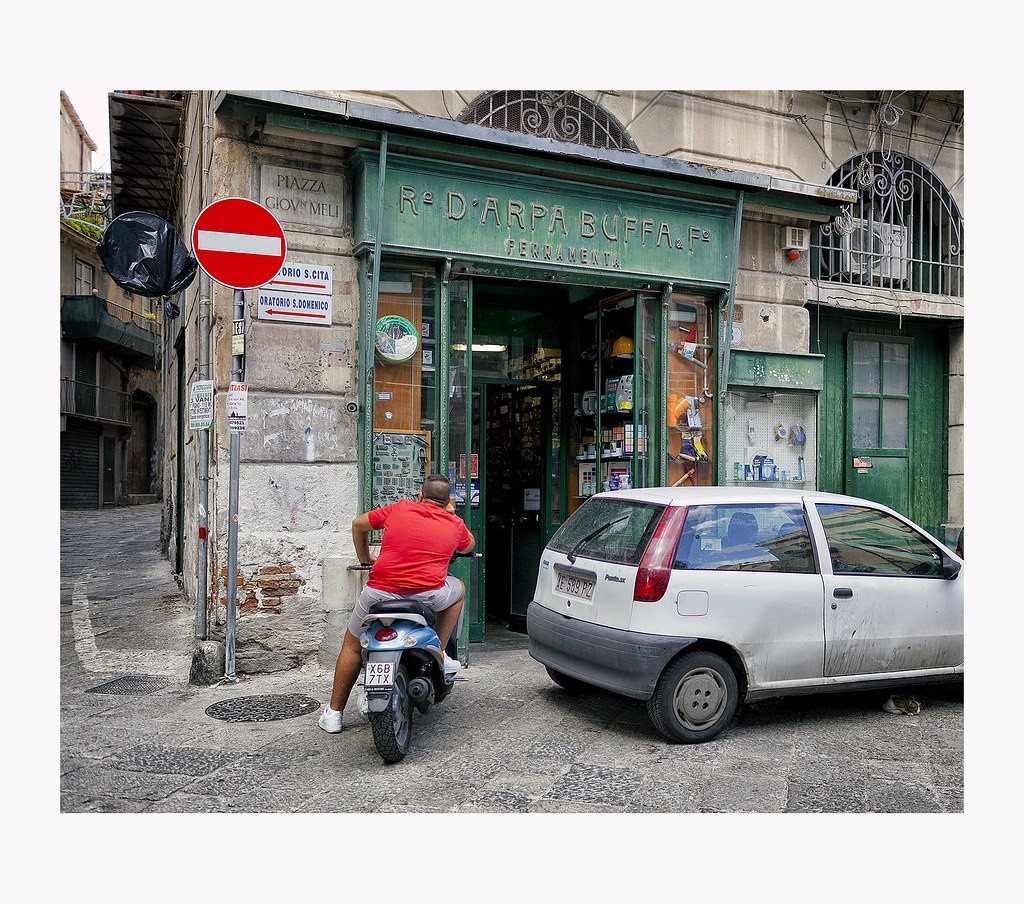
[
  {"left": 421, "top": 316, "right": 436, "bottom": 371},
  {"left": 572, "top": 354, "right": 648, "bottom": 500},
  {"left": 487, "top": 358, "right": 562, "bottom": 522}
]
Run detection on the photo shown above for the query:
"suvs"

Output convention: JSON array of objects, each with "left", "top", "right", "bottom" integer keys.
[{"left": 525, "top": 486, "right": 965, "bottom": 746}]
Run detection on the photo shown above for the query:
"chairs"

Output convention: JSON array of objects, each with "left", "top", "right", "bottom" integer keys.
[
  {"left": 776, "top": 522, "right": 795, "bottom": 554},
  {"left": 721, "top": 511, "right": 758, "bottom": 556}
]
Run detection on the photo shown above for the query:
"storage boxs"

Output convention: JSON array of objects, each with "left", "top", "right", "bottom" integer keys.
[{"left": 508, "top": 347, "right": 561, "bottom": 382}]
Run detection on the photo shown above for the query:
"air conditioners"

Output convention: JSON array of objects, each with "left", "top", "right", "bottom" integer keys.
[{"left": 820, "top": 216, "right": 909, "bottom": 284}]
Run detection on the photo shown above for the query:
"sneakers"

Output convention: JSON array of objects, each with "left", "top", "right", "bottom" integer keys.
[
  {"left": 318, "top": 703, "right": 343, "bottom": 733},
  {"left": 442, "top": 649, "right": 461, "bottom": 675}
]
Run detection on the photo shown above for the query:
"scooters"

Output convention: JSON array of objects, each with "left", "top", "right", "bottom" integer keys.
[{"left": 346, "top": 551, "right": 482, "bottom": 764}]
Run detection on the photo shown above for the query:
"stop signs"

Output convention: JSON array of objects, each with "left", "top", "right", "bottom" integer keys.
[{"left": 191, "top": 198, "right": 287, "bottom": 291}]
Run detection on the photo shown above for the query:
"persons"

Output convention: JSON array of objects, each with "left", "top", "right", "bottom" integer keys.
[{"left": 318, "top": 474, "right": 476, "bottom": 734}]
